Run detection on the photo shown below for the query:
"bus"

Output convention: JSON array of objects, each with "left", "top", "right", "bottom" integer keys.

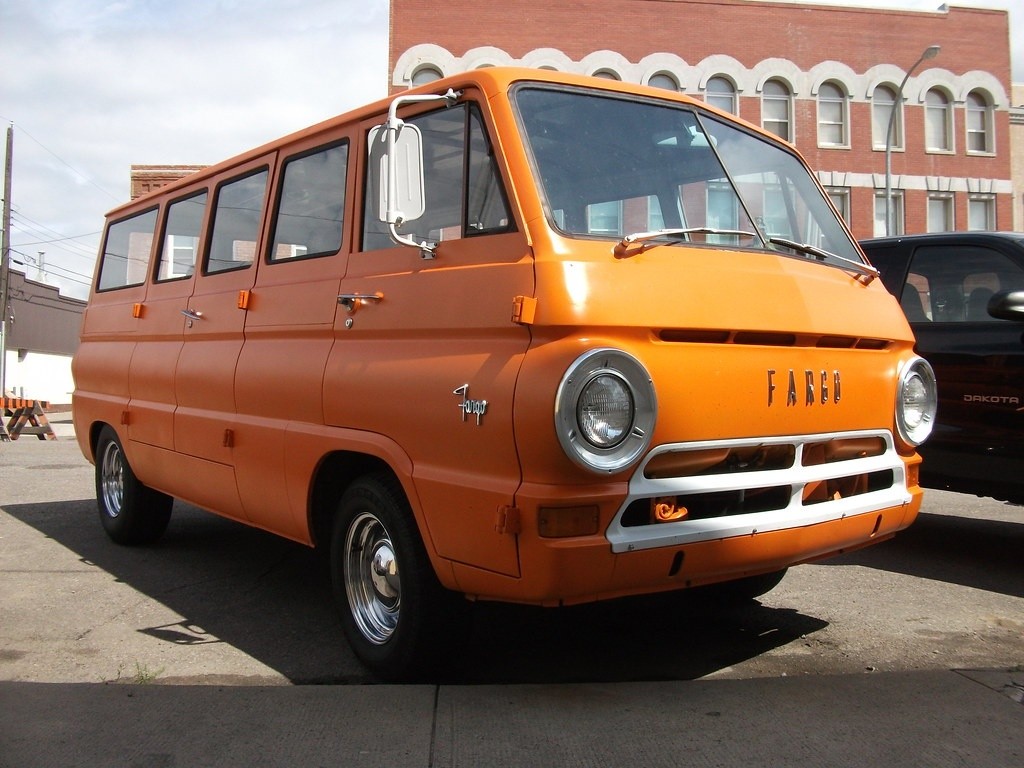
[{"left": 68, "top": 65, "right": 937, "bottom": 680}]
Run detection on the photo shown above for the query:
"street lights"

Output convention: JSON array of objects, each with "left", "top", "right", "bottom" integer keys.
[{"left": 883, "top": 44, "right": 939, "bottom": 238}]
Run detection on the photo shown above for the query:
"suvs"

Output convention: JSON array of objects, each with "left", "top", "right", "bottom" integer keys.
[{"left": 853, "top": 228, "right": 1023, "bottom": 514}]
